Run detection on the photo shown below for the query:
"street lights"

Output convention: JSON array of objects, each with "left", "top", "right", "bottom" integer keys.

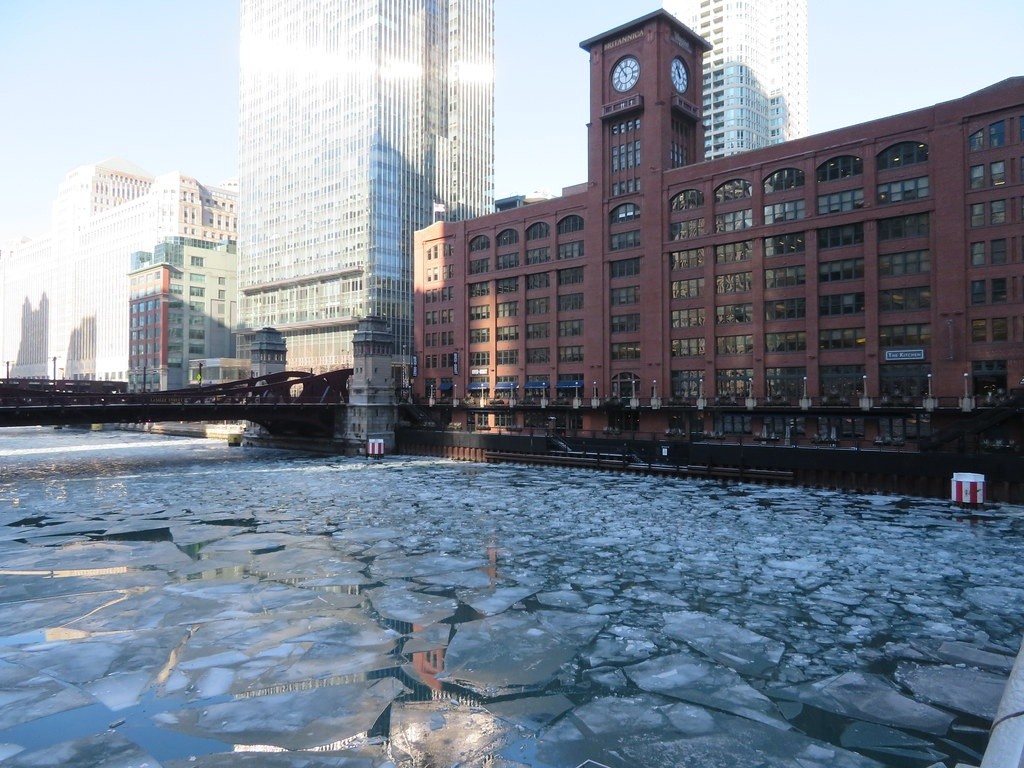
[
  {"left": 454, "top": 384, "right": 458, "bottom": 397},
  {"left": 748, "top": 378, "right": 754, "bottom": 398},
  {"left": 652, "top": 380, "right": 656, "bottom": 398},
  {"left": 510, "top": 383, "right": 512, "bottom": 398},
  {"left": 431, "top": 384, "right": 434, "bottom": 398},
  {"left": 804, "top": 376, "right": 807, "bottom": 396},
  {"left": 699, "top": 380, "right": 703, "bottom": 398},
  {"left": 962, "top": 372, "right": 970, "bottom": 398},
  {"left": 575, "top": 382, "right": 579, "bottom": 397},
  {"left": 862, "top": 376, "right": 868, "bottom": 398},
  {"left": 927, "top": 374, "right": 933, "bottom": 396},
  {"left": 481, "top": 382, "right": 484, "bottom": 399},
  {"left": 542, "top": 382, "right": 546, "bottom": 399},
  {"left": 632, "top": 381, "right": 636, "bottom": 397},
  {"left": 593, "top": 381, "right": 596, "bottom": 397}
]
